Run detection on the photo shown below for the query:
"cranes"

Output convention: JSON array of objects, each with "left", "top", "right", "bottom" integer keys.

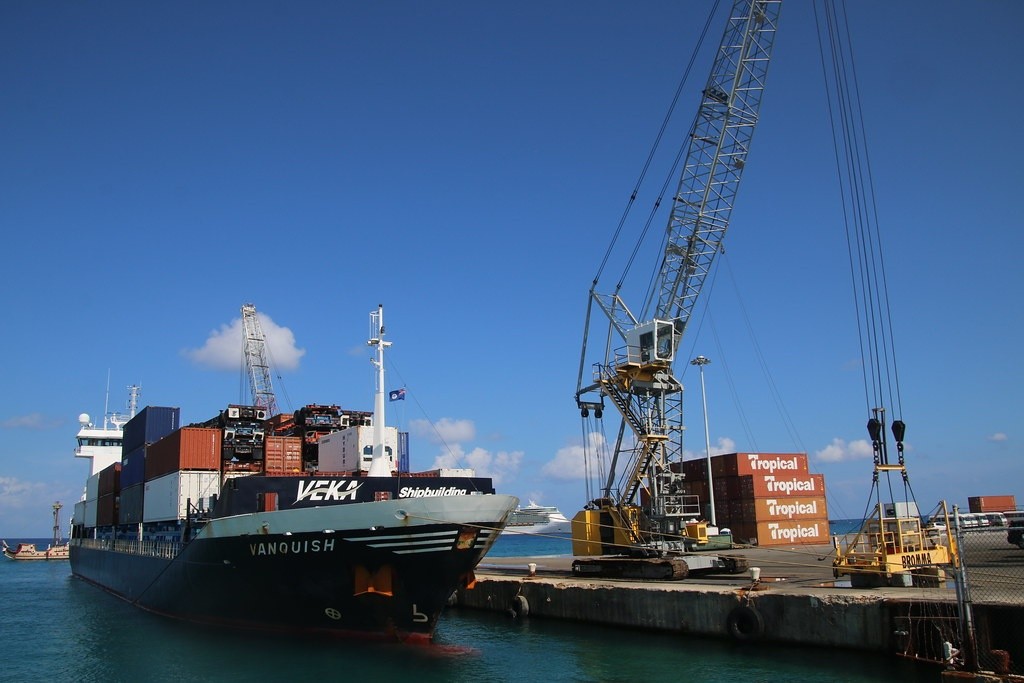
[{"left": 569, "top": 0, "right": 970, "bottom": 588}]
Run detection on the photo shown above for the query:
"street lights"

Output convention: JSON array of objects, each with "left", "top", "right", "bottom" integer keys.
[{"left": 690, "top": 356, "right": 717, "bottom": 525}]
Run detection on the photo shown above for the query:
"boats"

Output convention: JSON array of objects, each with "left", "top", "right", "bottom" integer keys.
[
  {"left": 69, "top": 301, "right": 520, "bottom": 642},
  {"left": 1, "top": 500, "right": 73, "bottom": 561},
  {"left": 498, "top": 502, "right": 573, "bottom": 535}
]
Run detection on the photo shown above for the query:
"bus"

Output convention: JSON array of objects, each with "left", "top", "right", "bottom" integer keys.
[
  {"left": 966, "top": 513, "right": 990, "bottom": 527},
  {"left": 986, "top": 512, "right": 1007, "bottom": 525},
  {"left": 928, "top": 514, "right": 972, "bottom": 529},
  {"left": 1003, "top": 510, "right": 1024, "bottom": 524}
]
